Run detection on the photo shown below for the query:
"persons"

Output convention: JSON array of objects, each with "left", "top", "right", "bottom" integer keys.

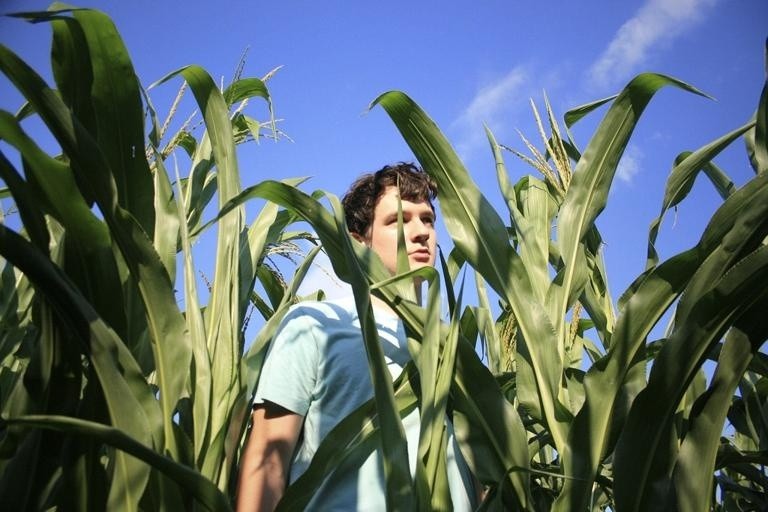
[{"left": 235, "top": 162, "right": 485, "bottom": 512}]
